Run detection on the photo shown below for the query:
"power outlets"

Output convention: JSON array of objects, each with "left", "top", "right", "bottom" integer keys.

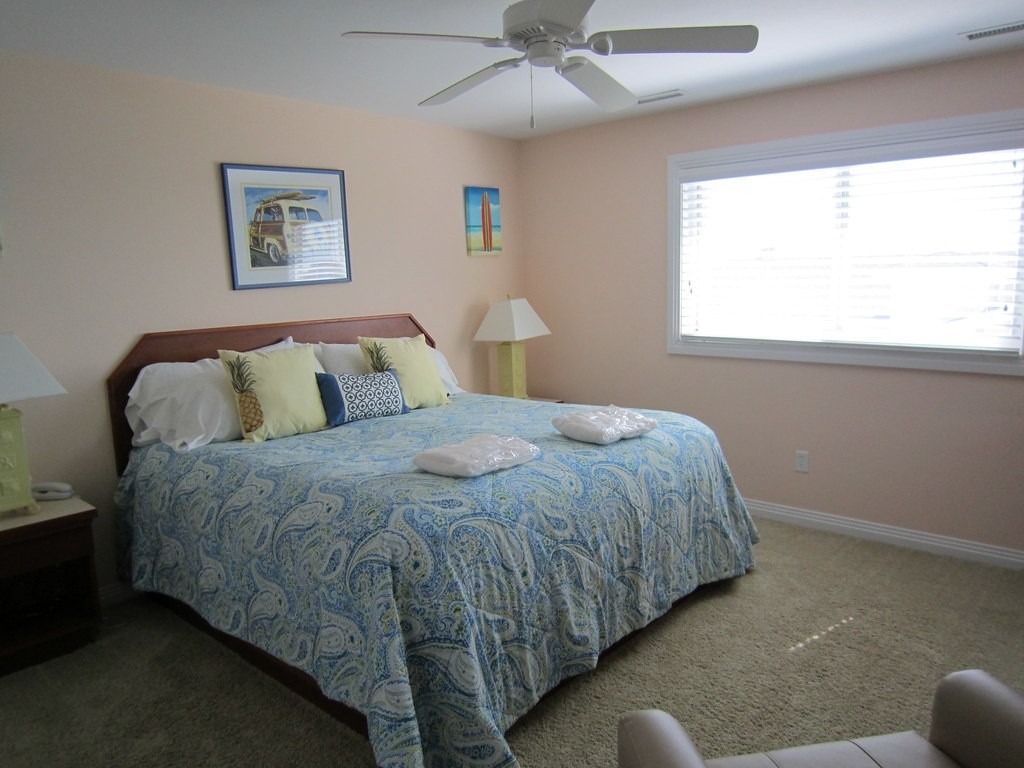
[{"left": 795, "top": 450, "right": 808, "bottom": 473}]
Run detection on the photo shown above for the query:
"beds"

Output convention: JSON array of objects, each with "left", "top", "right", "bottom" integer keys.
[{"left": 114, "top": 311, "right": 760, "bottom": 768}]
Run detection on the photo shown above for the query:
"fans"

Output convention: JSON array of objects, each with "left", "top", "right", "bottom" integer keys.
[{"left": 339, "top": 0, "right": 758, "bottom": 129}]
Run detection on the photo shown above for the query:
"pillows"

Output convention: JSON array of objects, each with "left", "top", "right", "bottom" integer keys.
[
  {"left": 318, "top": 339, "right": 465, "bottom": 397},
  {"left": 216, "top": 342, "right": 326, "bottom": 443},
  {"left": 357, "top": 334, "right": 450, "bottom": 409},
  {"left": 315, "top": 371, "right": 410, "bottom": 427},
  {"left": 139, "top": 344, "right": 326, "bottom": 451},
  {"left": 123, "top": 334, "right": 294, "bottom": 447}
]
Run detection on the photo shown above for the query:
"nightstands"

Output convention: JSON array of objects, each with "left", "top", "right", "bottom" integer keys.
[{"left": 0, "top": 496, "right": 99, "bottom": 677}]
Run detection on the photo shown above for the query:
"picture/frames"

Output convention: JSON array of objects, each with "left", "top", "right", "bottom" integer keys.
[{"left": 221, "top": 163, "right": 351, "bottom": 290}]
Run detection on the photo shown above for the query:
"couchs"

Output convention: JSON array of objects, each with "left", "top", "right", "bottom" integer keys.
[{"left": 618, "top": 664, "right": 1024, "bottom": 768}]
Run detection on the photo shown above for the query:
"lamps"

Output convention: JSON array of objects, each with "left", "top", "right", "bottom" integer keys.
[
  {"left": 0, "top": 333, "right": 68, "bottom": 518},
  {"left": 470, "top": 294, "right": 551, "bottom": 400}
]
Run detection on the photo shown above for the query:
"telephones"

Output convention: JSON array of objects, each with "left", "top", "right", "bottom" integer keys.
[{"left": 31, "top": 482, "right": 72, "bottom": 501}]
